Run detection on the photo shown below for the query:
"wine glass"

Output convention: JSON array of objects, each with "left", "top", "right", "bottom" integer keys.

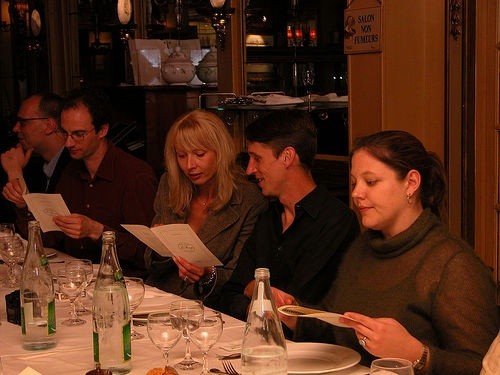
[
  {"left": 57, "top": 268, "right": 87, "bottom": 326},
  {"left": 123, "top": 277, "right": 145, "bottom": 340},
  {"left": 148, "top": 313, "right": 183, "bottom": 375},
  {"left": 169, "top": 299, "right": 204, "bottom": 369},
  {"left": 0, "top": 236, "right": 24, "bottom": 289},
  {"left": 0, "top": 223, "right": 16, "bottom": 274},
  {"left": 187, "top": 309, "right": 223, "bottom": 375},
  {"left": 302, "top": 63, "right": 316, "bottom": 113},
  {"left": 66, "top": 259, "right": 91, "bottom": 313}
]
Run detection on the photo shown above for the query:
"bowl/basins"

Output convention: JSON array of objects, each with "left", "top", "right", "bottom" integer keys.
[
  {"left": 196, "top": 46, "right": 216, "bottom": 86},
  {"left": 161, "top": 46, "right": 195, "bottom": 86}
]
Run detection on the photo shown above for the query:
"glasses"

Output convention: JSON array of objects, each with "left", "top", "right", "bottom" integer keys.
[
  {"left": 55, "top": 128, "right": 96, "bottom": 143},
  {"left": 15, "top": 117, "right": 48, "bottom": 125}
]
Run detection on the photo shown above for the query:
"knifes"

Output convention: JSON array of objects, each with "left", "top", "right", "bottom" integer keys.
[{"left": 131, "top": 313, "right": 170, "bottom": 318}]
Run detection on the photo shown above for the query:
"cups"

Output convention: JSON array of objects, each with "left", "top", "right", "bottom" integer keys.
[{"left": 370, "top": 357, "right": 415, "bottom": 375}]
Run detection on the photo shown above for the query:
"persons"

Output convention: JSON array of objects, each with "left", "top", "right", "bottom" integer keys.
[
  {"left": 270, "top": 129, "right": 500, "bottom": 375},
  {"left": 0, "top": 93, "right": 78, "bottom": 253},
  {"left": 207, "top": 107, "right": 361, "bottom": 341},
  {"left": 2, "top": 86, "right": 158, "bottom": 276},
  {"left": 143, "top": 110, "right": 265, "bottom": 312}
]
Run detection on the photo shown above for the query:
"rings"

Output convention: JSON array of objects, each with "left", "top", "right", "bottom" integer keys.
[
  {"left": 358, "top": 336, "right": 367, "bottom": 347},
  {"left": 183, "top": 276, "right": 189, "bottom": 282}
]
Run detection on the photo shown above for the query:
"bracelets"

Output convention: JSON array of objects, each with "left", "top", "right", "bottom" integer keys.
[{"left": 199, "top": 265, "right": 216, "bottom": 287}]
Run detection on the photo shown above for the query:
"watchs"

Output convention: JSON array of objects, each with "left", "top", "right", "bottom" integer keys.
[{"left": 413, "top": 344, "right": 428, "bottom": 372}]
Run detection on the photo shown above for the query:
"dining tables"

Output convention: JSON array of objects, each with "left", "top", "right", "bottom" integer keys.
[{"left": 0, "top": 232, "right": 396, "bottom": 375}]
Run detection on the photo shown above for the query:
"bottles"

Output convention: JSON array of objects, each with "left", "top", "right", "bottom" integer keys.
[
  {"left": 91, "top": 231, "right": 133, "bottom": 375},
  {"left": 20, "top": 220, "right": 57, "bottom": 351},
  {"left": 240, "top": 268, "right": 287, "bottom": 375}
]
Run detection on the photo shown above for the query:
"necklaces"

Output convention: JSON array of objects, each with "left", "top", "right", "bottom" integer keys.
[{"left": 202, "top": 201, "right": 207, "bottom": 207}]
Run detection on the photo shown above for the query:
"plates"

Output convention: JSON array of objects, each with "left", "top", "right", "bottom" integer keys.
[
  {"left": 21, "top": 239, "right": 56, "bottom": 262},
  {"left": 91, "top": 263, "right": 100, "bottom": 280},
  {"left": 87, "top": 284, "right": 199, "bottom": 317},
  {"left": 286, "top": 343, "right": 362, "bottom": 375}
]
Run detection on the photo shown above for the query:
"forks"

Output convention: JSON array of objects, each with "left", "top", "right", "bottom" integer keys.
[{"left": 222, "top": 360, "right": 240, "bottom": 375}]
